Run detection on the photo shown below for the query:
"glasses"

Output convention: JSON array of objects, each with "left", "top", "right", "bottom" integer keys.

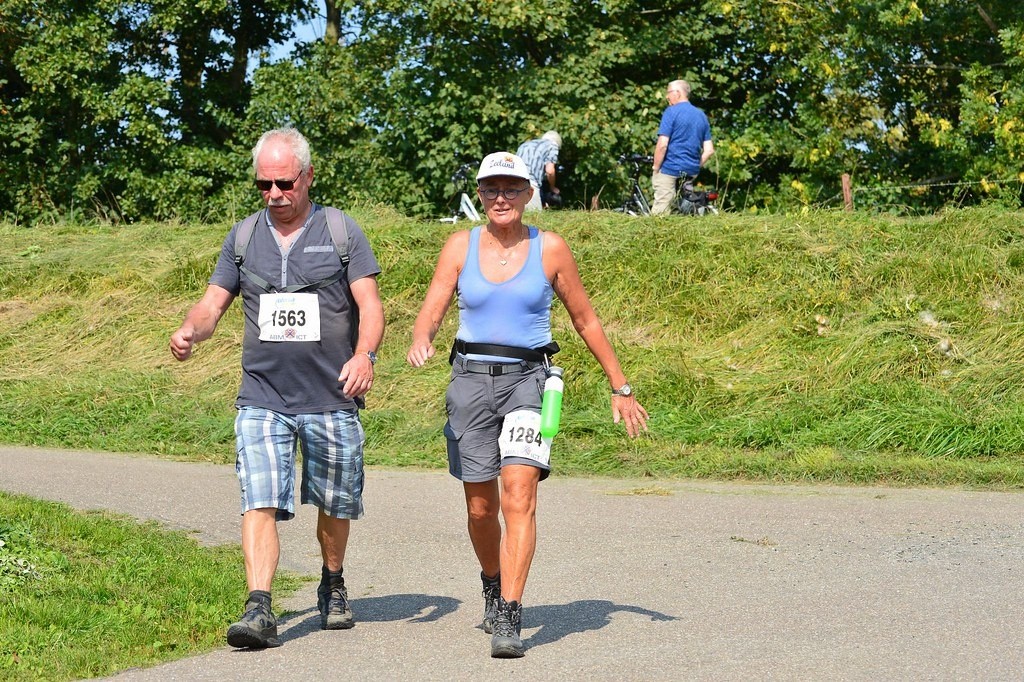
[
  {"left": 254, "top": 170, "right": 302, "bottom": 190},
  {"left": 481, "top": 187, "right": 530, "bottom": 200}
]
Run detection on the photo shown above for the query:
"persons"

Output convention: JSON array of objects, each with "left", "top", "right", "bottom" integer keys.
[
  {"left": 515, "top": 130, "right": 562, "bottom": 211},
  {"left": 651, "top": 80, "right": 715, "bottom": 216},
  {"left": 168, "top": 128, "right": 386, "bottom": 648},
  {"left": 406, "top": 152, "right": 651, "bottom": 657}
]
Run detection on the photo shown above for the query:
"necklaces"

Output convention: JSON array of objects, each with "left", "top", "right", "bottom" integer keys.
[{"left": 487, "top": 223, "right": 524, "bottom": 266}]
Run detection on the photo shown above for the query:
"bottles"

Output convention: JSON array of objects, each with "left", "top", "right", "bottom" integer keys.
[{"left": 541, "top": 366, "right": 565, "bottom": 437}]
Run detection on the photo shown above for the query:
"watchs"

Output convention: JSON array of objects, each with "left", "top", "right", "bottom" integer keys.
[
  {"left": 355, "top": 350, "right": 377, "bottom": 365},
  {"left": 604, "top": 381, "right": 634, "bottom": 400}
]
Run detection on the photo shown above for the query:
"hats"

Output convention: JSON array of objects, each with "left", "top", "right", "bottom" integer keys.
[{"left": 475, "top": 152, "right": 531, "bottom": 186}]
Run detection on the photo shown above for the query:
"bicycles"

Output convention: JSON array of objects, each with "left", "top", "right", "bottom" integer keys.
[{"left": 612, "top": 152, "right": 720, "bottom": 217}]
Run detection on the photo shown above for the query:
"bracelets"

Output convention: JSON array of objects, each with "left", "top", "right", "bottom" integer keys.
[{"left": 652, "top": 166, "right": 659, "bottom": 171}]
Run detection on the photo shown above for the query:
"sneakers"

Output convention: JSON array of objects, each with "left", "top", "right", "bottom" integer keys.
[
  {"left": 227, "top": 597, "right": 280, "bottom": 647},
  {"left": 491, "top": 596, "right": 525, "bottom": 657},
  {"left": 316, "top": 581, "right": 355, "bottom": 630},
  {"left": 481, "top": 570, "right": 501, "bottom": 634}
]
